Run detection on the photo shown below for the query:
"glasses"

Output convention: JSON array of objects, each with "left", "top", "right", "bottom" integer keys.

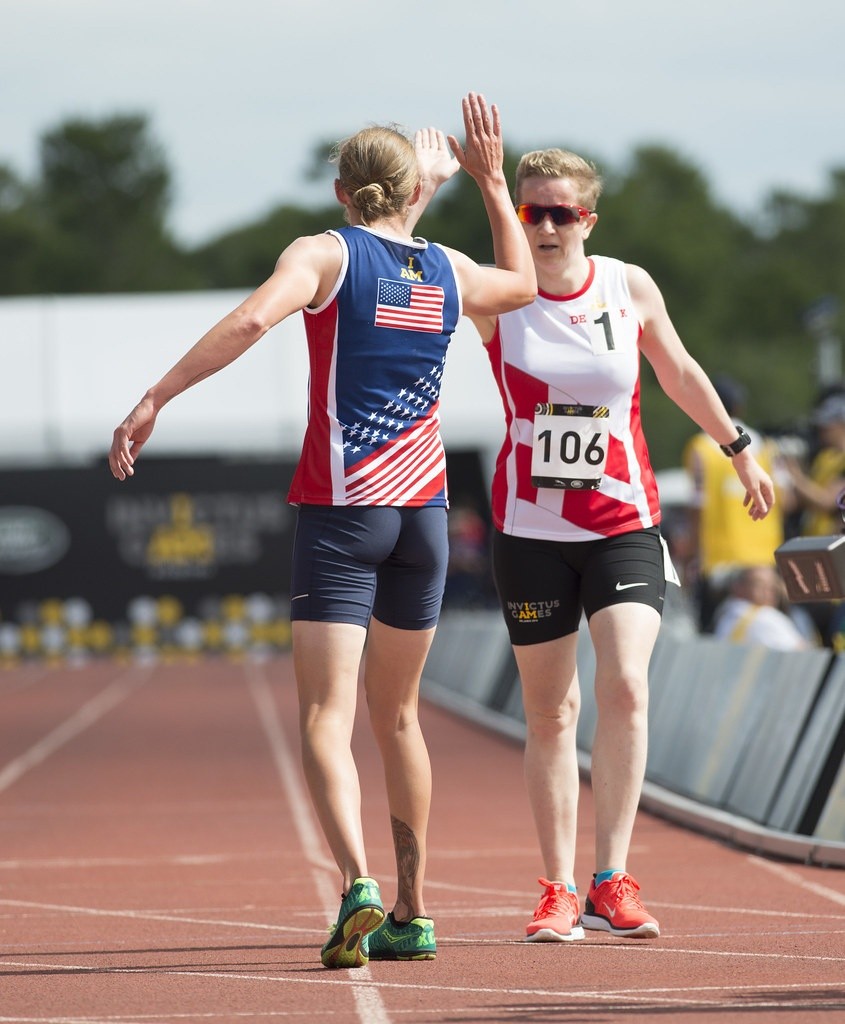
[{"left": 515, "top": 203, "right": 592, "bottom": 225}]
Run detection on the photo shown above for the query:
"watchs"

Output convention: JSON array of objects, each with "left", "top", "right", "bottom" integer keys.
[{"left": 720, "top": 426, "right": 753, "bottom": 457}]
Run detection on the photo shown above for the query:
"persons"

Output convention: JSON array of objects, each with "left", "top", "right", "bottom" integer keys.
[
  {"left": 685, "top": 376, "right": 845, "bottom": 651},
  {"left": 110, "top": 93, "right": 541, "bottom": 969},
  {"left": 403, "top": 125, "right": 775, "bottom": 943}
]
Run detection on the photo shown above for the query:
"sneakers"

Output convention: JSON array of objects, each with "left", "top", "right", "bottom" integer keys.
[
  {"left": 527, "top": 878, "right": 585, "bottom": 942},
  {"left": 320, "top": 876, "right": 386, "bottom": 969},
  {"left": 581, "top": 871, "right": 661, "bottom": 938},
  {"left": 369, "top": 911, "right": 437, "bottom": 959}
]
